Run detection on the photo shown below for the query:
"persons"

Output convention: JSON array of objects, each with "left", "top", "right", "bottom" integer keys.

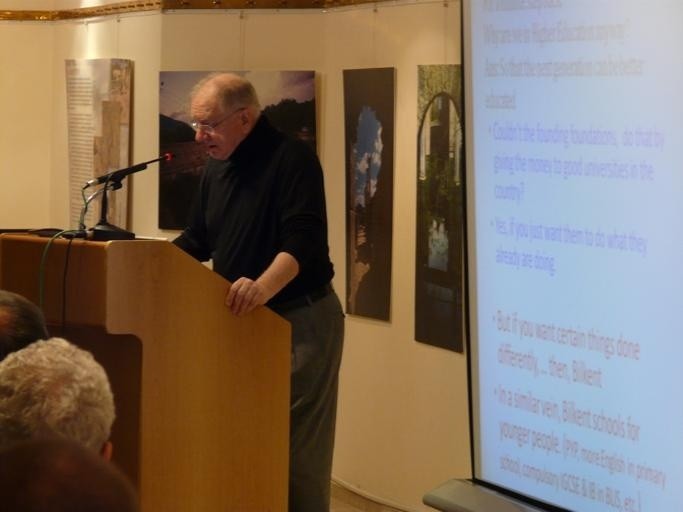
[
  {"left": 170, "top": 71, "right": 345, "bottom": 512},
  {"left": 0, "top": 288, "right": 146, "bottom": 512}
]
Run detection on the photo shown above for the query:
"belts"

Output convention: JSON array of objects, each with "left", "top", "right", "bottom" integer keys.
[{"left": 268, "top": 283, "right": 335, "bottom": 315}]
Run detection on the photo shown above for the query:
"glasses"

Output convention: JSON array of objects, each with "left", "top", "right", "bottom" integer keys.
[{"left": 191, "top": 107, "right": 249, "bottom": 135}]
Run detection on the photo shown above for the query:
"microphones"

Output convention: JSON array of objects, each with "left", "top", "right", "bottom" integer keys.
[{"left": 90, "top": 152, "right": 175, "bottom": 240}]
